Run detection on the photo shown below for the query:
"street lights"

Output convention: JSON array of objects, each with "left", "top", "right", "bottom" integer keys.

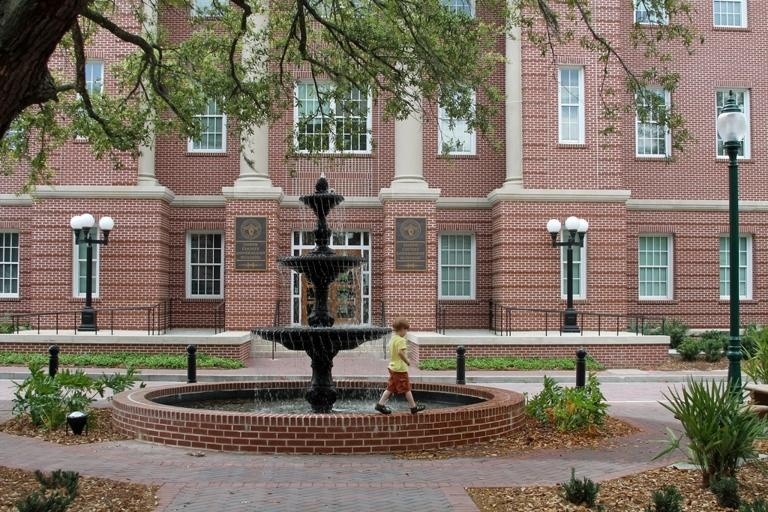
[
  {"left": 70, "top": 213, "right": 114, "bottom": 332},
  {"left": 716, "top": 88, "right": 749, "bottom": 409},
  {"left": 546, "top": 216, "right": 589, "bottom": 333}
]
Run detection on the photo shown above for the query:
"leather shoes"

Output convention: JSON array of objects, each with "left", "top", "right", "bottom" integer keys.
[
  {"left": 411, "top": 405, "right": 425, "bottom": 413},
  {"left": 375, "top": 404, "right": 391, "bottom": 414}
]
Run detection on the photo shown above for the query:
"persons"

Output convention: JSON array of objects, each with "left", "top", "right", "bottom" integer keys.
[{"left": 375, "top": 317, "right": 425, "bottom": 414}]
